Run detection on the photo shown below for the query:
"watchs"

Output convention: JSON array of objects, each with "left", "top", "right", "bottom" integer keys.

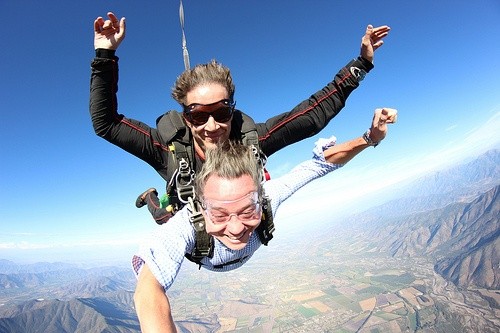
[{"left": 362, "top": 130, "right": 378, "bottom": 148}]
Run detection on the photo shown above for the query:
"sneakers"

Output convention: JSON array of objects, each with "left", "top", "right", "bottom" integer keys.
[{"left": 136, "top": 187, "right": 158, "bottom": 208}]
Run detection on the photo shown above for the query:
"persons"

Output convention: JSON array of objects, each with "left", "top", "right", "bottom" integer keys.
[{"left": 90, "top": 12, "right": 397, "bottom": 333}]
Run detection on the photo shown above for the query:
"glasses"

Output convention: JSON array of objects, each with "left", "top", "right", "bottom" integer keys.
[
  {"left": 180, "top": 98, "right": 235, "bottom": 126},
  {"left": 196, "top": 190, "right": 263, "bottom": 224}
]
